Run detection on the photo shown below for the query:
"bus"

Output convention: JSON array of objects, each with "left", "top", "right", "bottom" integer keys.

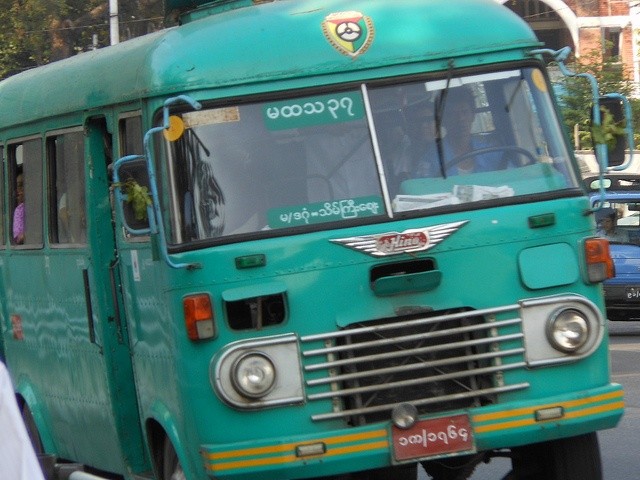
[{"left": 0, "top": 0, "right": 634, "bottom": 480}]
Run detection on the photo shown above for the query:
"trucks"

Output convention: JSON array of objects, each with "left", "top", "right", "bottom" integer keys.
[{"left": 582, "top": 172, "right": 640, "bottom": 322}]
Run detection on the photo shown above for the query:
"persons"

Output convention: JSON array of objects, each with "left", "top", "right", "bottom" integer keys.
[
  {"left": 387, "top": 101, "right": 442, "bottom": 183},
  {"left": 590, "top": 203, "right": 630, "bottom": 244},
  {"left": 9, "top": 167, "right": 54, "bottom": 247},
  {"left": 415, "top": 92, "right": 515, "bottom": 178}
]
